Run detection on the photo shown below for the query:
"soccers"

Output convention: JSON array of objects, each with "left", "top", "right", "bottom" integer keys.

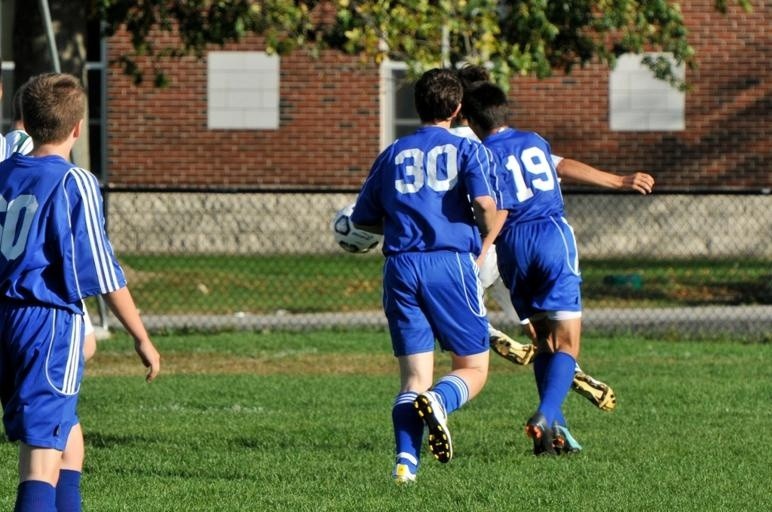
[{"left": 334, "top": 204, "right": 382, "bottom": 254}]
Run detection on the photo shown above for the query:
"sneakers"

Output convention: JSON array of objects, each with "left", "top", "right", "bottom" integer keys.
[
  {"left": 489, "top": 329, "right": 539, "bottom": 366},
  {"left": 570, "top": 370, "right": 617, "bottom": 412},
  {"left": 526, "top": 414, "right": 555, "bottom": 456},
  {"left": 393, "top": 463, "right": 416, "bottom": 485},
  {"left": 553, "top": 425, "right": 582, "bottom": 455},
  {"left": 413, "top": 391, "right": 454, "bottom": 464}
]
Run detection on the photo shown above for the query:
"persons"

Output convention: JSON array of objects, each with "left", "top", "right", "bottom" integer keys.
[
  {"left": 0, "top": 78, "right": 36, "bottom": 161},
  {"left": 350, "top": 68, "right": 497, "bottom": 484},
  {"left": 447, "top": 63, "right": 655, "bottom": 412},
  {"left": 462, "top": 83, "right": 582, "bottom": 454},
  {"left": 0, "top": 73, "right": 161, "bottom": 511}
]
